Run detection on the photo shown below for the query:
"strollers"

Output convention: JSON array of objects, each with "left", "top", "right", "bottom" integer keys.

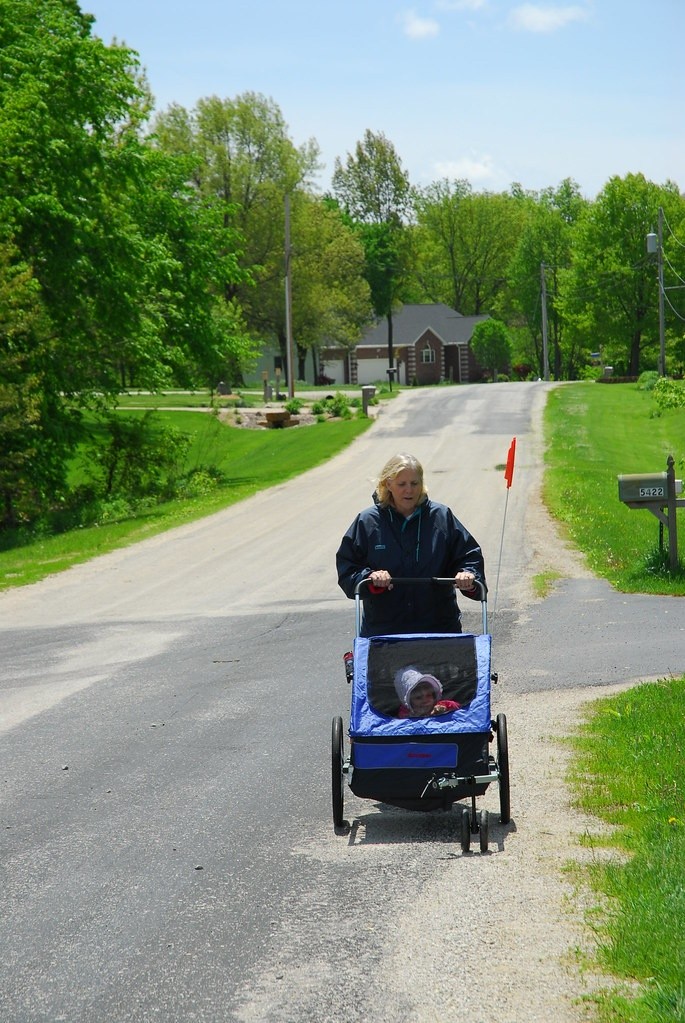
[{"left": 330, "top": 574, "right": 512, "bottom": 854}]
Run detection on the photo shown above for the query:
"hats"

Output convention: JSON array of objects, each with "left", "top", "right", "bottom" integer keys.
[{"left": 394, "top": 665, "right": 443, "bottom": 715}]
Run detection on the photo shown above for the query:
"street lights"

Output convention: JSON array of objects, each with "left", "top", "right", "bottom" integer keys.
[{"left": 646, "top": 207, "right": 667, "bottom": 379}]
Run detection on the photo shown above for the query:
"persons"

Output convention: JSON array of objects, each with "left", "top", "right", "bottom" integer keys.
[
  {"left": 393, "top": 664, "right": 462, "bottom": 717},
  {"left": 336, "top": 454, "right": 488, "bottom": 637}
]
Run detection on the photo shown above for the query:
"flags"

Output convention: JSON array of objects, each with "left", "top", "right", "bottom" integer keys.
[{"left": 505, "top": 437, "right": 516, "bottom": 489}]
